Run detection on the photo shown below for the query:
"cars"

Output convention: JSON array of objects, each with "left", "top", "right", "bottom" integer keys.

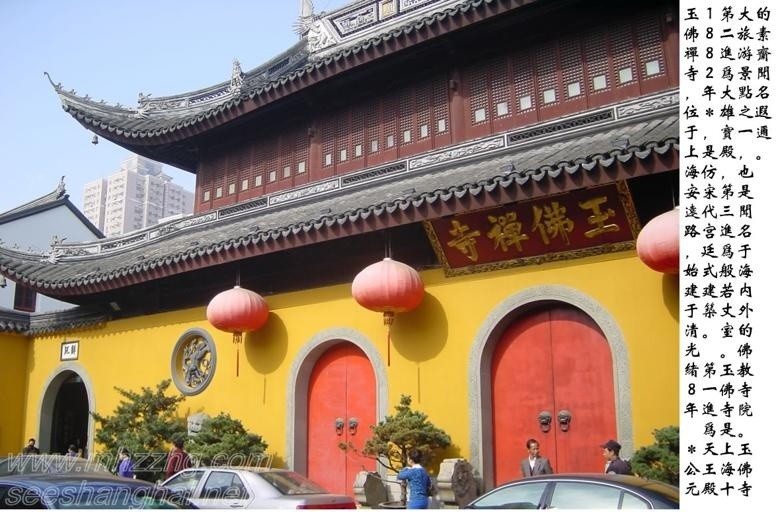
[
  {"left": 158, "top": 467, "right": 356, "bottom": 509},
  {"left": 0, "top": 474, "right": 198, "bottom": 509},
  {"left": 463, "top": 473, "right": 679, "bottom": 509},
  {"left": 1, "top": 454, "right": 114, "bottom": 478}
]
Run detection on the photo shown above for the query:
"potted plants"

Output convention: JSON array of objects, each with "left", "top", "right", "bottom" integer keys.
[{"left": 336, "top": 395, "right": 453, "bottom": 510}]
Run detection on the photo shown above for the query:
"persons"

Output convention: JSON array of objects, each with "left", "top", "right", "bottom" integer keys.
[
  {"left": 65, "top": 444, "right": 78, "bottom": 458},
  {"left": 165, "top": 438, "right": 191, "bottom": 480},
  {"left": 396, "top": 449, "right": 432, "bottom": 509},
  {"left": 600, "top": 439, "right": 630, "bottom": 473},
  {"left": 521, "top": 439, "right": 552, "bottom": 478},
  {"left": 22, "top": 438, "right": 40, "bottom": 455},
  {"left": 117, "top": 447, "right": 134, "bottom": 478}
]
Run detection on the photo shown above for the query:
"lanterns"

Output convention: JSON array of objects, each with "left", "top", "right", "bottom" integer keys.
[
  {"left": 351, "top": 258, "right": 424, "bottom": 325},
  {"left": 207, "top": 286, "right": 269, "bottom": 343},
  {"left": 636, "top": 206, "right": 680, "bottom": 275}
]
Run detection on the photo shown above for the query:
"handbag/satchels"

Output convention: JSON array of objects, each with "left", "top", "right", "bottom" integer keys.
[{"left": 425, "top": 471, "right": 439, "bottom": 498}]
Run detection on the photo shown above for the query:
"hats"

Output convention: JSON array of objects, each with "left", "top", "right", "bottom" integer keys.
[{"left": 599, "top": 439, "right": 622, "bottom": 456}]
